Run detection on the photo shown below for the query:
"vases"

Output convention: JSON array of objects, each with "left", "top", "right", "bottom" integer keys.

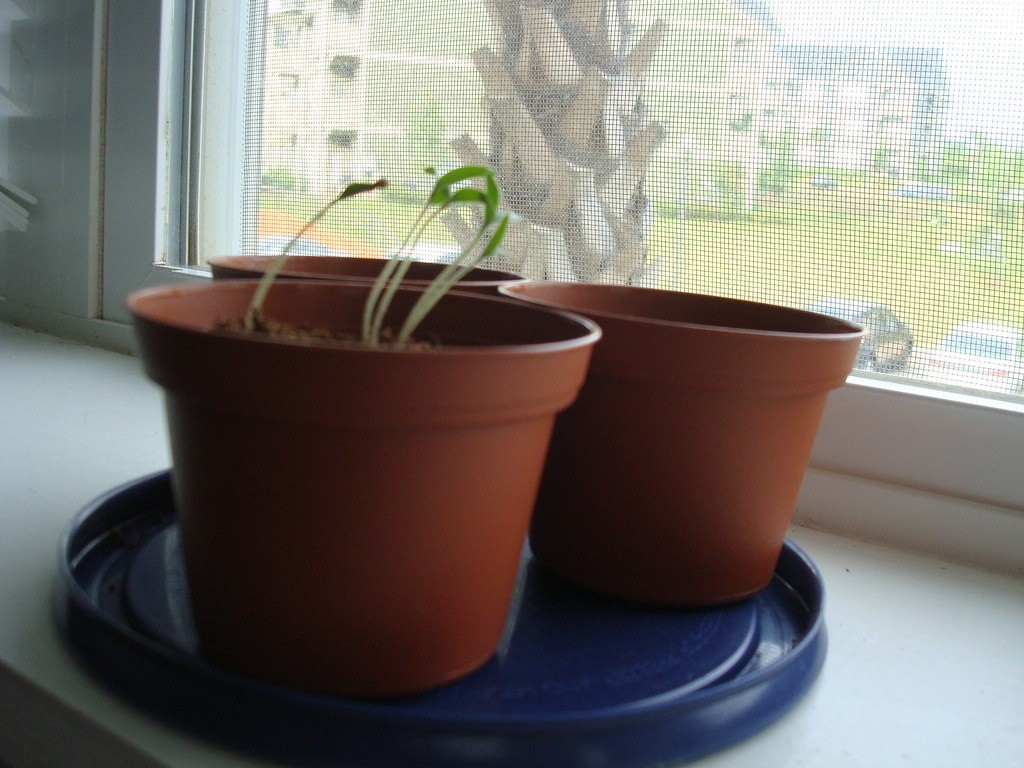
[
  {"left": 206, "top": 254, "right": 525, "bottom": 291},
  {"left": 496, "top": 281, "right": 867, "bottom": 609}
]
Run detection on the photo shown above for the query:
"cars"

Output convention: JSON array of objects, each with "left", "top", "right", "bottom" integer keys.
[
  {"left": 385, "top": 240, "right": 476, "bottom": 267},
  {"left": 932, "top": 319, "right": 1024, "bottom": 397},
  {"left": 805, "top": 295, "right": 915, "bottom": 372},
  {"left": 257, "top": 235, "right": 348, "bottom": 256}
]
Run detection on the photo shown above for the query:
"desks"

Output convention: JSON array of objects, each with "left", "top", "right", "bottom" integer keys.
[{"left": 0, "top": 319, "right": 1024, "bottom": 768}]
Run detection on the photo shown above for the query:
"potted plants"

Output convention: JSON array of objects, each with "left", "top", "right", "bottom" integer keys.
[{"left": 127, "top": 167, "right": 600, "bottom": 699}]
